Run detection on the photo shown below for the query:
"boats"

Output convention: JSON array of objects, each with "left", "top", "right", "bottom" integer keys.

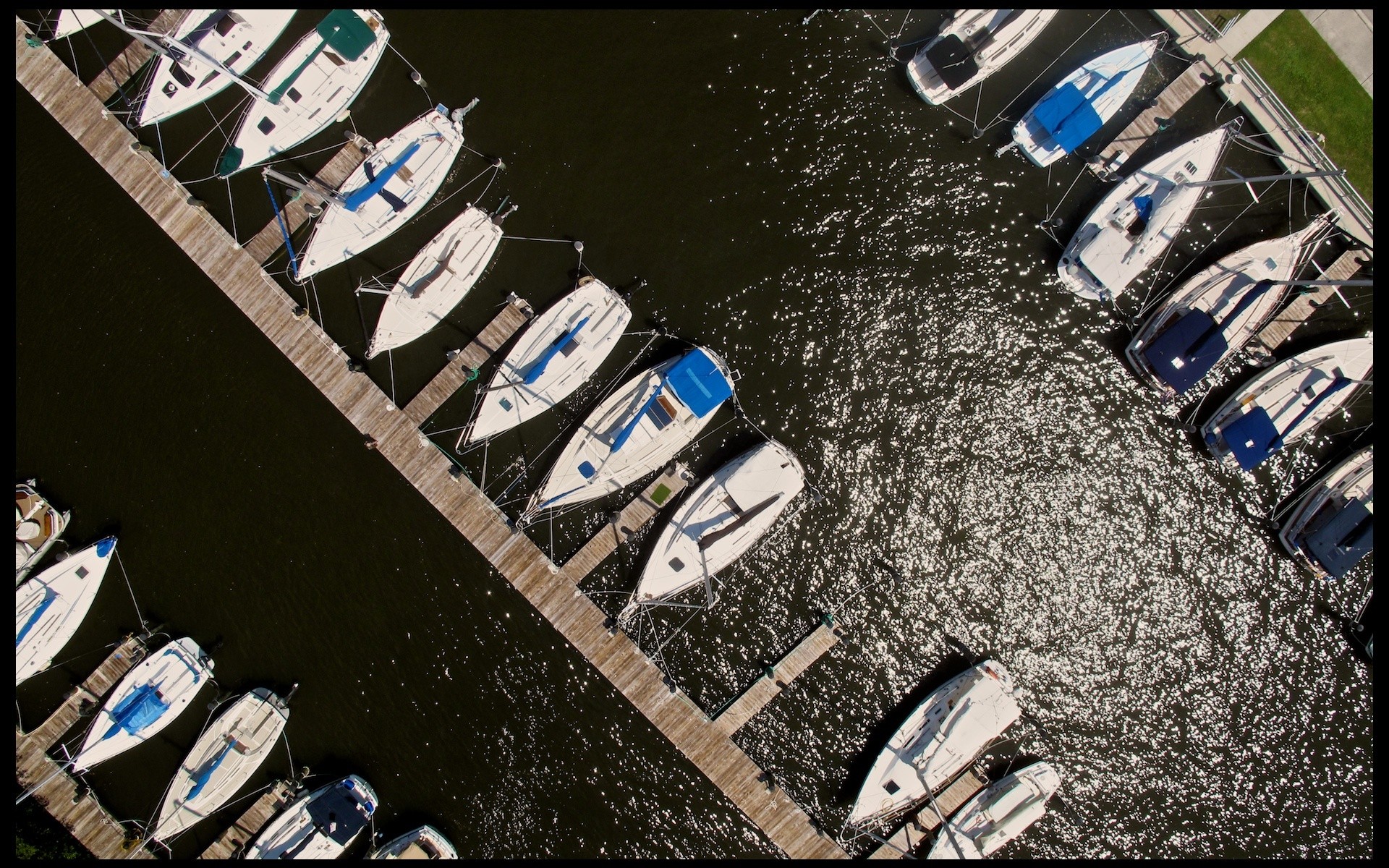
[
  {"left": 996, "top": 27, "right": 1172, "bottom": 167},
  {"left": 608, "top": 436, "right": 818, "bottom": 628},
  {"left": 14, "top": 535, "right": 119, "bottom": 688},
  {"left": 46, "top": 635, "right": 217, "bottom": 779},
  {"left": 12, "top": 479, "right": 75, "bottom": 591},
  {"left": 1265, "top": 444, "right": 1376, "bottom": 584},
  {"left": 455, "top": 277, "right": 638, "bottom": 459},
  {"left": 243, "top": 774, "right": 379, "bottom": 860},
  {"left": 509, "top": 345, "right": 746, "bottom": 531},
  {"left": 924, "top": 758, "right": 1064, "bottom": 861},
  {"left": 836, "top": 661, "right": 1022, "bottom": 847},
  {"left": 905, "top": 8, "right": 1058, "bottom": 105},
  {"left": 19, "top": 7, "right": 121, "bottom": 47},
  {"left": 1124, "top": 208, "right": 1343, "bottom": 394},
  {"left": 367, "top": 824, "right": 462, "bottom": 861},
  {"left": 359, "top": 196, "right": 521, "bottom": 362},
  {"left": 1196, "top": 336, "right": 1378, "bottom": 477},
  {"left": 213, "top": 7, "right": 393, "bottom": 187},
  {"left": 121, "top": 6, "right": 300, "bottom": 135},
  {"left": 1053, "top": 107, "right": 1246, "bottom": 302},
  {"left": 133, "top": 681, "right": 303, "bottom": 854},
  {"left": 287, "top": 96, "right": 486, "bottom": 287}
]
